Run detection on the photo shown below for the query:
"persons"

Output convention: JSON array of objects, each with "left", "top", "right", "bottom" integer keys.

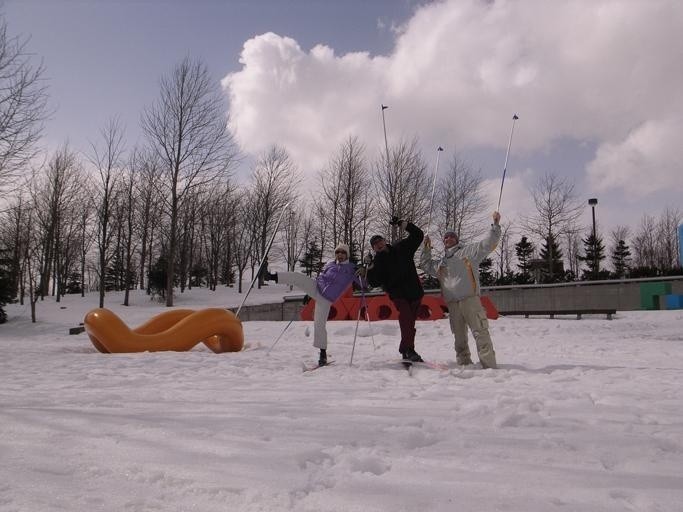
[
  {"left": 363, "top": 215, "right": 426, "bottom": 362},
  {"left": 257, "top": 243, "right": 370, "bottom": 366},
  {"left": 416, "top": 210, "right": 503, "bottom": 369}
]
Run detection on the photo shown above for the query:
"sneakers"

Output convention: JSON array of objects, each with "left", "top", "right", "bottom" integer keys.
[
  {"left": 260, "top": 262, "right": 270, "bottom": 278},
  {"left": 319, "top": 353, "right": 327, "bottom": 366},
  {"left": 402, "top": 348, "right": 422, "bottom": 361}
]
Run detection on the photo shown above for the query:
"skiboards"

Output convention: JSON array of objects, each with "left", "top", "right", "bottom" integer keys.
[
  {"left": 236, "top": 200, "right": 334, "bottom": 370},
  {"left": 383, "top": 358, "right": 466, "bottom": 377}
]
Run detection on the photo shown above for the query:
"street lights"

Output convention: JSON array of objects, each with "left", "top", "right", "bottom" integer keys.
[{"left": 588, "top": 199, "right": 598, "bottom": 280}]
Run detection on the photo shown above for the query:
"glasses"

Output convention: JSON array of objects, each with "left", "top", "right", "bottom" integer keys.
[{"left": 335, "top": 250, "right": 347, "bottom": 255}]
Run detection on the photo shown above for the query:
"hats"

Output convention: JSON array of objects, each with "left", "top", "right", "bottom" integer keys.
[
  {"left": 442, "top": 232, "right": 458, "bottom": 242},
  {"left": 336, "top": 243, "right": 350, "bottom": 257},
  {"left": 370, "top": 234, "right": 382, "bottom": 247}
]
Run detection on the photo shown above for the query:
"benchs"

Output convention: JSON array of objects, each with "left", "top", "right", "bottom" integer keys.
[{"left": 498, "top": 309, "right": 617, "bottom": 321}]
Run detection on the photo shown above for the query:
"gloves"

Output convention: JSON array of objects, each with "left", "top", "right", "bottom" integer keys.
[
  {"left": 492, "top": 211, "right": 501, "bottom": 223},
  {"left": 358, "top": 268, "right": 365, "bottom": 277},
  {"left": 391, "top": 216, "right": 405, "bottom": 226},
  {"left": 423, "top": 235, "right": 430, "bottom": 245},
  {"left": 364, "top": 254, "right": 371, "bottom": 264}
]
